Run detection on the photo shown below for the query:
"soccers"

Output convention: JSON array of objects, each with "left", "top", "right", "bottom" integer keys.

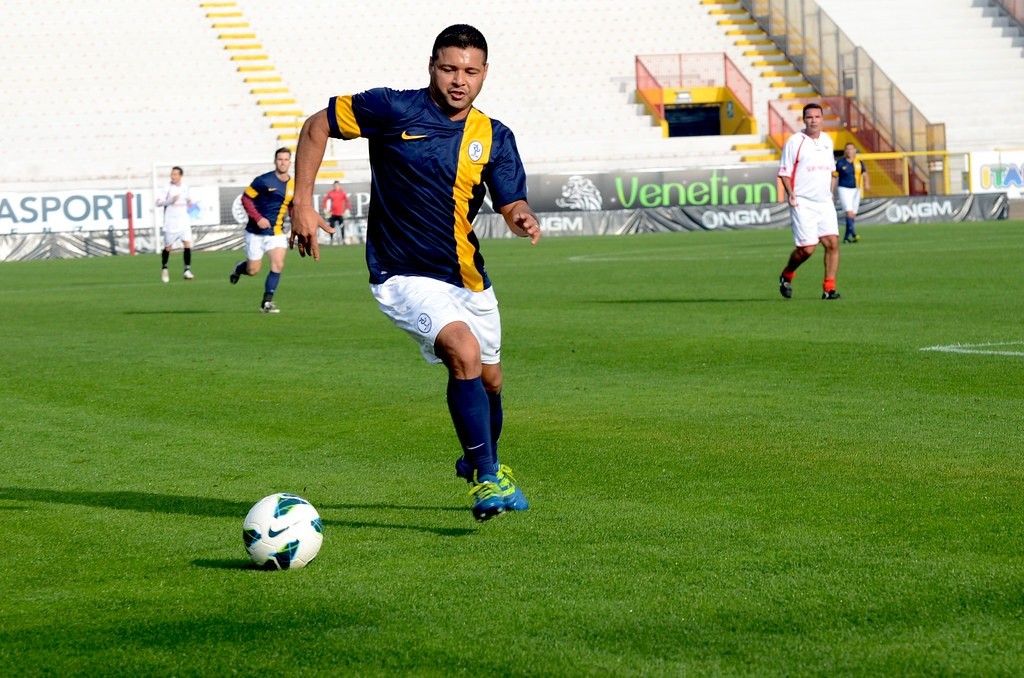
[{"left": 242, "top": 491, "right": 324, "bottom": 570}]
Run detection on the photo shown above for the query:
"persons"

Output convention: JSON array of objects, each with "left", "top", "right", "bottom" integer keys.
[
  {"left": 777, "top": 103, "right": 840, "bottom": 299},
  {"left": 230, "top": 148, "right": 294, "bottom": 313},
  {"left": 156, "top": 166, "right": 194, "bottom": 282},
  {"left": 289, "top": 24, "right": 541, "bottom": 521},
  {"left": 323, "top": 181, "right": 351, "bottom": 246},
  {"left": 836, "top": 142, "right": 869, "bottom": 243}
]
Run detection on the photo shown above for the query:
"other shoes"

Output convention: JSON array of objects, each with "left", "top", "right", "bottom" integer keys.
[
  {"left": 852, "top": 235, "right": 859, "bottom": 243},
  {"left": 844, "top": 237, "right": 850, "bottom": 243},
  {"left": 184, "top": 271, "right": 193, "bottom": 278},
  {"left": 161, "top": 267, "right": 170, "bottom": 283}
]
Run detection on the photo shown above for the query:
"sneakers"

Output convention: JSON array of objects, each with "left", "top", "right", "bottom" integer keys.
[
  {"left": 229, "top": 260, "right": 242, "bottom": 284},
  {"left": 259, "top": 299, "right": 281, "bottom": 313},
  {"left": 780, "top": 274, "right": 792, "bottom": 298},
  {"left": 466, "top": 467, "right": 504, "bottom": 521},
  {"left": 822, "top": 290, "right": 841, "bottom": 299},
  {"left": 455, "top": 453, "right": 528, "bottom": 510}
]
